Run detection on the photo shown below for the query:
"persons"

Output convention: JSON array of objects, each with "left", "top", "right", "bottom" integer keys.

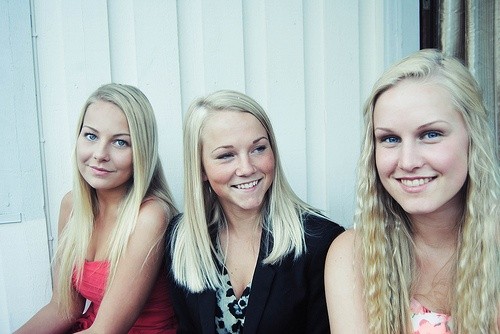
[
  {"left": 325, "top": 48, "right": 500, "bottom": 333},
  {"left": 12, "top": 82, "right": 182, "bottom": 334},
  {"left": 158, "top": 89, "right": 345, "bottom": 334}
]
